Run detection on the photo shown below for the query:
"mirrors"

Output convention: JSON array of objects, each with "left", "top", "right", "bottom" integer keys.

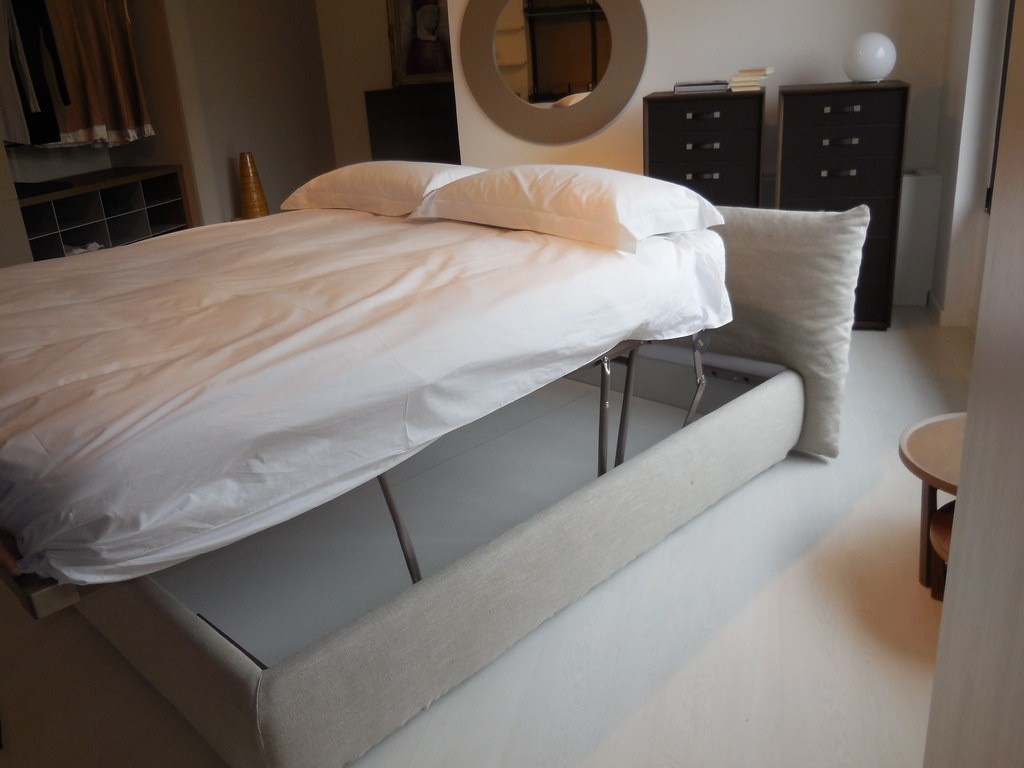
[{"left": 459, "top": 0, "right": 649, "bottom": 148}]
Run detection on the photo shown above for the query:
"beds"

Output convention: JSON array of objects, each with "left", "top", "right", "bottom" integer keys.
[{"left": 0, "top": 158, "right": 871, "bottom": 768}]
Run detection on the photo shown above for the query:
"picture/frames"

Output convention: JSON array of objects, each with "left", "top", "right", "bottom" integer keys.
[{"left": 386, "top": 0, "right": 453, "bottom": 86}]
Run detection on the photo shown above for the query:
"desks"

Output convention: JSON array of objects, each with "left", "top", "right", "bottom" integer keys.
[{"left": 898, "top": 413, "right": 967, "bottom": 602}]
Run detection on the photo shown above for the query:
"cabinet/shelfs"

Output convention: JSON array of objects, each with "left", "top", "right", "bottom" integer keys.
[
  {"left": 18, "top": 164, "right": 194, "bottom": 262},
  {"left": 777, "top": 79, "right": 911, "bottom": 331},
  {"left": 641, "top": 86, "right": 765, "bottom": 208}
]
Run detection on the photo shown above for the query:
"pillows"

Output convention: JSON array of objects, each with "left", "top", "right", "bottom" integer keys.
[
  {"left": 656, "top": 204, "right": 872, "bottom": 460},
  {"left": 279, "top": 161, "right": 490, "bottom": 217},
  {"left": 406, "top": 163, "right": 725, "bottom": 254}
]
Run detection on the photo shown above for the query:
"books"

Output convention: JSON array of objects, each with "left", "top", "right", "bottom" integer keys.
[
  {"left": 674, "top": 80, "right": 729, "bottom": 94},
  {"left": 729, "top": 68, "right": 771, "bottom": 92}
]
